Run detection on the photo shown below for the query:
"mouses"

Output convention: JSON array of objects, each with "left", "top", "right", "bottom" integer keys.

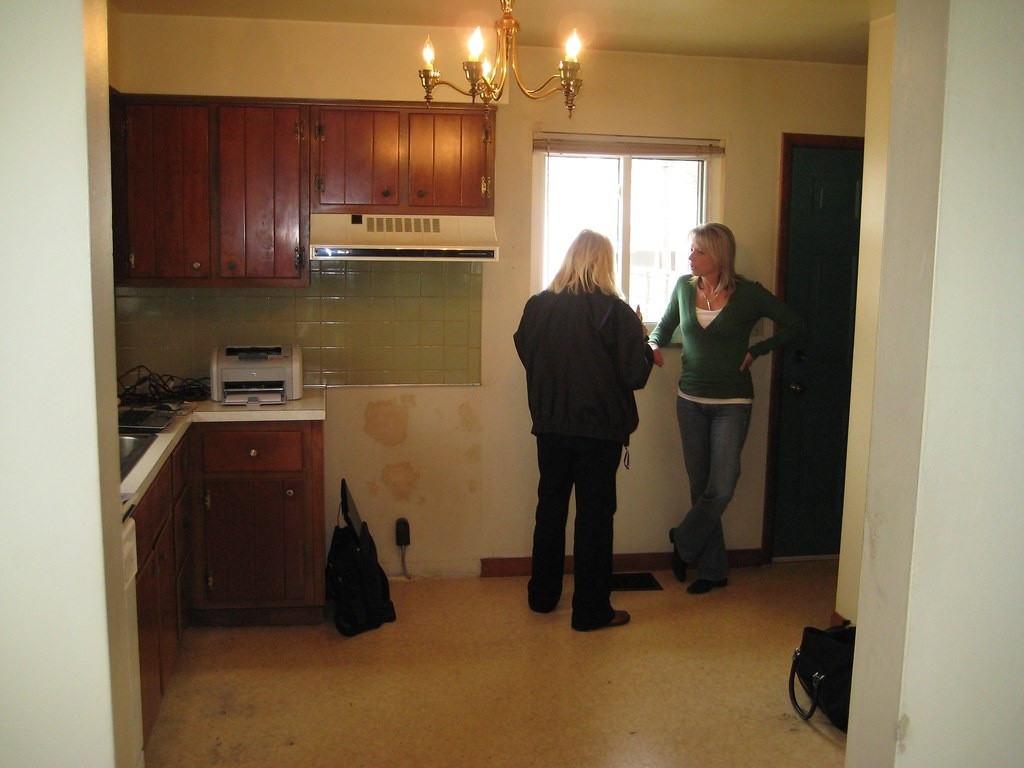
[{"left": 157, "top": 399, "right": 182, "bottom": 410}]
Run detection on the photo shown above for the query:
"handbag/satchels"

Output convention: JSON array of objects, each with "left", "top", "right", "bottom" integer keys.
[
  {"left": 789, "top": 619, "right": 855, "bottom": 733},
  {"left": 326, "top": 478, "right": 397, "bottom": 635}
]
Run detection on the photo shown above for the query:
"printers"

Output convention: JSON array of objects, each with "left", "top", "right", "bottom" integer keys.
[{"left": 210, "top": 344, "right": 303, "bottom": 407}]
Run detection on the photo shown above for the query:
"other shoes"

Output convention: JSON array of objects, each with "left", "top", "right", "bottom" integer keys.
[
  {"left": 670, "top": 527, "right": 687, "bottom": 581},
  {"left": 606, "top": 610, "right": 630, "bottom": 627},
  {"left": 688, "top": 578, "right": 727, "bottom": 593}
]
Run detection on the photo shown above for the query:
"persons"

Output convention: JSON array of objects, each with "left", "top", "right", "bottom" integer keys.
[
  {"left": 514, "top": 229, "right": 655, "bottom": 632},
  {"left": 648, "top": 224, "right": 805, "bottom": 595}
]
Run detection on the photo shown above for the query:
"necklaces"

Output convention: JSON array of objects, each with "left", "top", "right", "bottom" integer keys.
[{"left": 702, "top": 284, "right": 721, "bottom": 311}]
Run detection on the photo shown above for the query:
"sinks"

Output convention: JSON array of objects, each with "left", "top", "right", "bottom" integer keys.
[{"left": 119, "top": 432, "right": 158, "bottom": 483}]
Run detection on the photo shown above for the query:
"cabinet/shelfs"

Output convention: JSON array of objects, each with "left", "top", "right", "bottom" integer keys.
[
  {"left": 107, "top": 86, "right": 497, "bottom": 289},
  {"left": 131, "top": 421, "right": 328, "bottom": 750}
]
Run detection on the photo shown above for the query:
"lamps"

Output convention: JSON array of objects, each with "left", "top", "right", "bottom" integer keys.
[{"left": 419, "top": 0, "right": 584, "bottom": 122}]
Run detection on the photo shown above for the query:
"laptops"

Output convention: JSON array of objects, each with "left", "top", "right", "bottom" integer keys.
[{"left": 118, "top": 410, "right": 176, "bottom": 431}]
